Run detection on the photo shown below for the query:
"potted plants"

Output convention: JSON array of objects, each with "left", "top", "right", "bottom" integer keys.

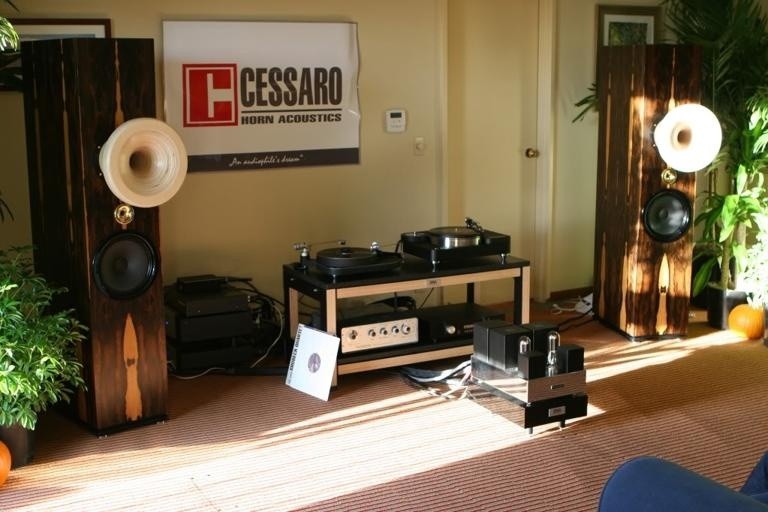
[
  {"left": 0, "top": 242, "right": 90, "bottom": 470},
  {"left": 669, "top": 0, "right": 767, "bottom": 332}
]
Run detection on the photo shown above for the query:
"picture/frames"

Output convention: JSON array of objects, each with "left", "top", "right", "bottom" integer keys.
[{"left": 596, "top": 5, "right": 663, "bottom": 47}]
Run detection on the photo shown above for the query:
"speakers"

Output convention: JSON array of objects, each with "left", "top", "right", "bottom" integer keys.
[
  {"left": 594, "top": 45, "right": 723, "bottom": 341},
  {"left": 19, "top": 37, "right": 189, "bottom": 438}
]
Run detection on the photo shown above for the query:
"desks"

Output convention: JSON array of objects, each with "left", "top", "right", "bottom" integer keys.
[{"left": 284, "top": 255, "right": 532, "bottom": 393}]
